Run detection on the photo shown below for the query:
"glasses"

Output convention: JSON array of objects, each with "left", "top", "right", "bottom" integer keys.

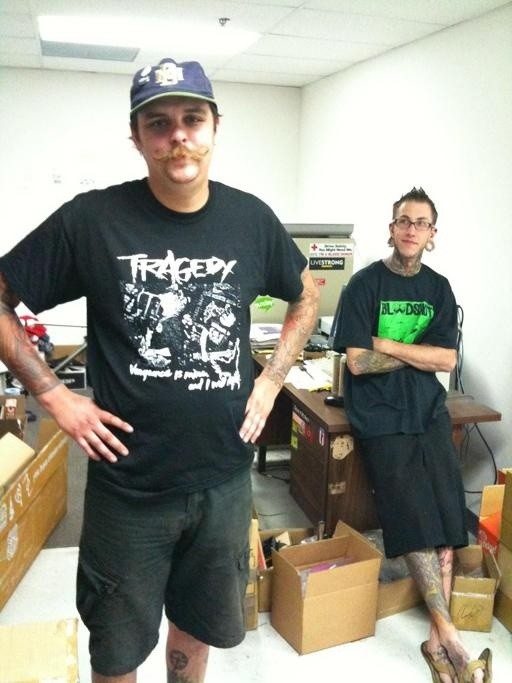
[{"left": 390, "top": 215, "right": 435, "bottom": 232}]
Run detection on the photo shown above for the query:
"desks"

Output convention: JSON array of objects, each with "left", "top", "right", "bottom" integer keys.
[{"left": 251, "top": 350, "right": 501, "bottom": 537}]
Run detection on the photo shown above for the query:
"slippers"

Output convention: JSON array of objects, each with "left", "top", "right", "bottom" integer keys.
[
  {"left": 421, "top": 638, "right": 457, "bottom": 682},
  {"left": 458, "top": 648, "right": 492, "bottom": 683}
]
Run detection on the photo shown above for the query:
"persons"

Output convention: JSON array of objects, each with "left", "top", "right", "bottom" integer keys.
[
  {"left": 334, "top": 186, "right": 491, "bottom": 683},
  {"left": 0, "top": 60, "right": 319, "bottom": 682}
]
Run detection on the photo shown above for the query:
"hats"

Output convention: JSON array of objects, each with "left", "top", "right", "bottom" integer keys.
[{"left": 130, "top": 59, "right": 216, "bottom": 125}]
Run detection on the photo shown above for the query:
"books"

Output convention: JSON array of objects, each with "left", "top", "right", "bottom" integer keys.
[{"left": 248, "top": 323, "right": 284, "bottom": 350}]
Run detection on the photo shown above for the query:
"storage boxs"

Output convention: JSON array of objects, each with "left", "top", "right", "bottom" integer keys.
[
  {"left": 494, "top": 468, "right": 512, "bottom": 635},
  {"left": 1, "top": 394, "right": 70, "bottom": 611},
  {"left": 271, "top": 521, "right": 381, "bottom": 653},
  {"left": 44, "top": 345, "right": 87, "bottom": 391},
  {"left": 259, "top": 525, "right": 317, "bottom": 613},
  {"left": 452, "top": 545, "right": 500, "bottom": 632}
]
